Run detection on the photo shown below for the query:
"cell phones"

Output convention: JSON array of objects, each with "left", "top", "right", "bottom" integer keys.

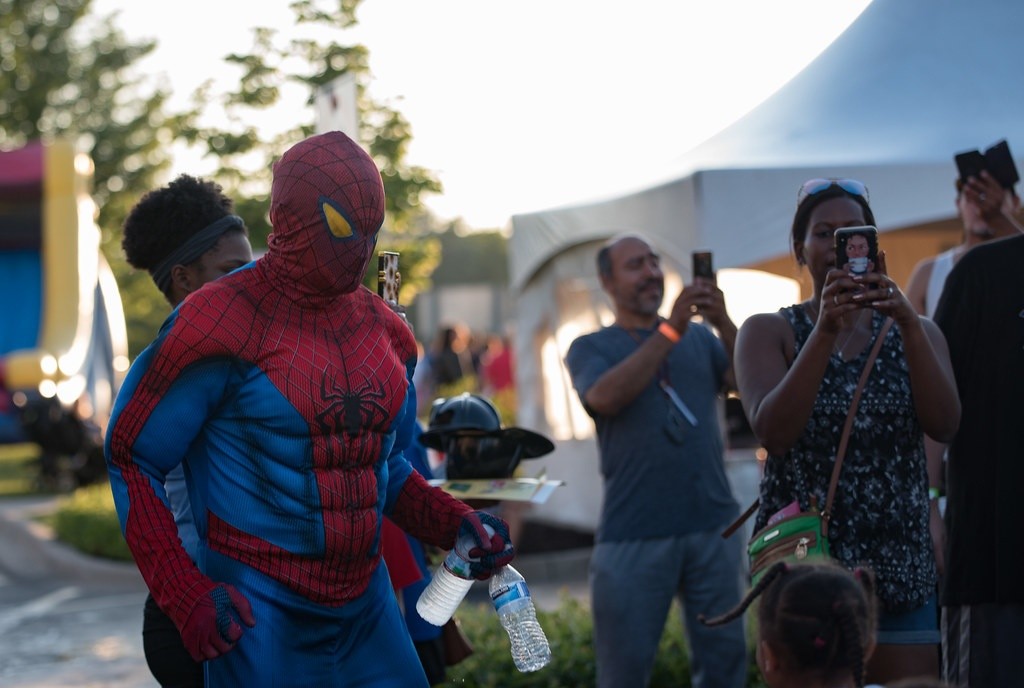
[
  {"left": 956, "top": 151, "right": 983, "bottom": 183},
  {"left": 835, "top": 226, "right": 879, "bottom": 305},
  {"left": 694, "top": 253, "right": 713, "bottom": 289},
  {"left": 378, "top": 252, "right": 399, "bottom": 308}
]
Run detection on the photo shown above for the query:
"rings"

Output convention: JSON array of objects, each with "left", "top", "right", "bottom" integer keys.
[
  {"left": 888, "top": 287, "right": 894, "bottom": 299},
  {"left": 834, "top": 296, "right": 839, "bottom": 305},
  {"left": 977, "top": 192, "right": 985, "bottom": 204}
]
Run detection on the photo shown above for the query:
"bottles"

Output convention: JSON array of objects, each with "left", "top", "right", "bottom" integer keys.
[
  {"left": 490, "top": 563, "right": 551, "bottom": 673},
  {"left": 416, "top": 523, "right": 495, "bottom": 626}
]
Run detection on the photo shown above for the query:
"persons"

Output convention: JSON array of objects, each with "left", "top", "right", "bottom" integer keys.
[
  {"left": 103, "top": 130, "right": 515, "bottom": 688},
  {"left": 564, "top": 235, "right": 750, "bottom": 688},
  {"left": 905, "top": 167, "right": 1024, "bottom": 688},
  {"left": 698, "top": 560, "right": 884, "bottom": 688},
  {"left": 735, "top": 179, "right": 961, "bottom": 688}
]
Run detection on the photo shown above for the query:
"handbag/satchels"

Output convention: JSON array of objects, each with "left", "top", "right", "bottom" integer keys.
[{"left": 747, "top": 511, "right": 831, "bottom": 588}]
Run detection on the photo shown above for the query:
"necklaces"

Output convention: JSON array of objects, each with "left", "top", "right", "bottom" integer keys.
[{"left": 808, "top": 300, "right": 864, "bottom": 360}]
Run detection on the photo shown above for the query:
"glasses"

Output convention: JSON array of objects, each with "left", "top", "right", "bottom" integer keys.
[{"left": 798, "top": 179, "right": 869, "bottom": 205}]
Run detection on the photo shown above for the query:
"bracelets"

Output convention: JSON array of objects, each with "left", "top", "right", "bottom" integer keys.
[
  {"left": 929, "top": 487, "right": 939, "bottom": 500},
  {"left": 658, "top": 321, "right": 681, "bottom": 344}
]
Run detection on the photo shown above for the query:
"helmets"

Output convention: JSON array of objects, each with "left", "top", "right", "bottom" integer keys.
[{"left": 418, "top": 392, "right": 504, "bottom": 456}]
